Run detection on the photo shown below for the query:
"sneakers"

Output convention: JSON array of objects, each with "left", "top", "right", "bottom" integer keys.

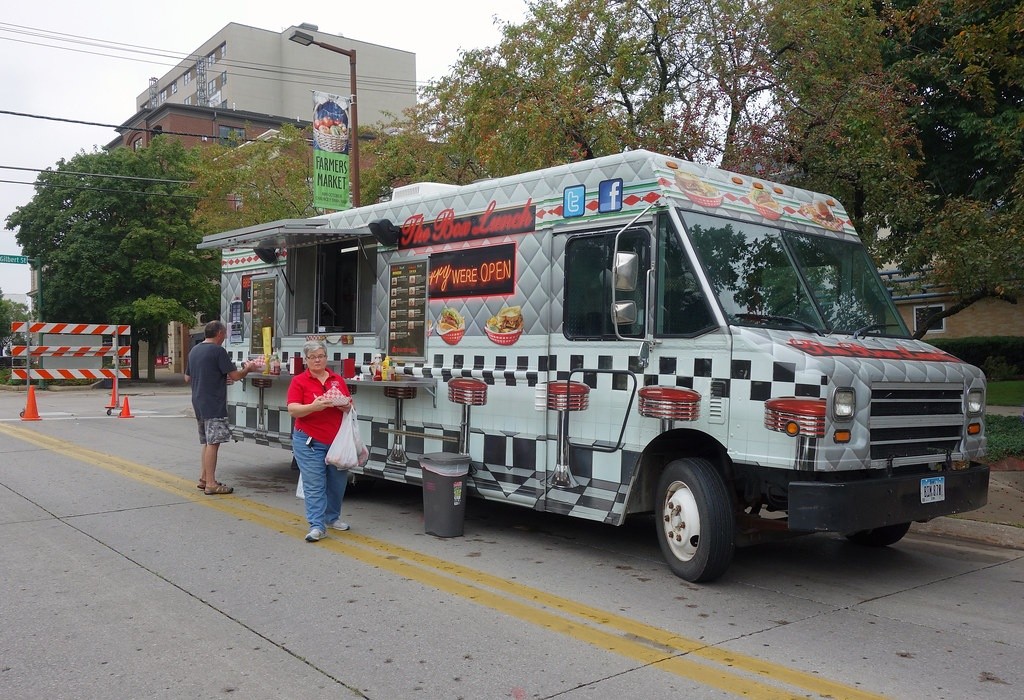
[
  {"left": 324, "top": 518, "right": 350, "bottom": 531},
  {"left": 305, "top": 529, "right": 327, "bottom": 541}
]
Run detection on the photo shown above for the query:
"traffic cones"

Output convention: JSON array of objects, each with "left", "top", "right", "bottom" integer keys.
[
  {"left": 20, "top": 385, "right": 42, "bottom": 421},
  {"left": 119, "top": 396, "right": 134, "bottom": 418}
]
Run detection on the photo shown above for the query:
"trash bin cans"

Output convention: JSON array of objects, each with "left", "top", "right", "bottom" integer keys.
[{"left": 418, "top": 452, "right": 473, "bottom": 538}]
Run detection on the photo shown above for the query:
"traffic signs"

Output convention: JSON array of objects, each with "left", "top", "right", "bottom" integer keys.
[{"left": 0, "top": 254, "right": 28, "bottom": 264}]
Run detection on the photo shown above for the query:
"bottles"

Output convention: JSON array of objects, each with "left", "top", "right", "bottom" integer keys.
[
  {"left": 270, "top": 352, "right": 280, "bottom": 375},
  {"left": 370, "top": 354, "right": 400, "bottom": 381},
  {"left": 262, "top": 353, "right": 270, "bottom": 374}
]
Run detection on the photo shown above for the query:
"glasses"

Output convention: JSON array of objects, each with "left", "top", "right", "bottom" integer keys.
[{"left": 306, "top": 355, "right": 326, "bottom": 359}]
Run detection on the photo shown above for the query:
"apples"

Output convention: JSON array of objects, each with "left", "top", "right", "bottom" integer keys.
[{"left": 315, "top": 116, "right": 347, "bottom": 137}]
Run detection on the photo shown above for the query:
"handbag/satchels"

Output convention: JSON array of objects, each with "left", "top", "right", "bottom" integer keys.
[
  {"left": 351, "top": 406, "right": 369, "bottom": 466},
  {"left": 324, "top": 405, "right": 358, "bottom": 471}
]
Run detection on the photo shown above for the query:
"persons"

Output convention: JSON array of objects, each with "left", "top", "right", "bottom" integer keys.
[
  {"left": 286, "top": 340, "right": 356, "bottom": 540},
  {"left": 183, "top": 321, "right": 257, "bottom": 495}
]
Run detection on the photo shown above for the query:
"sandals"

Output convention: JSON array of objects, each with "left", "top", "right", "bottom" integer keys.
[
  {"left": 197, "top": 478, "right": 226, "bottom": 490},
  {"left": 204, "top": 484, "right": 233, "bottom": 495}
]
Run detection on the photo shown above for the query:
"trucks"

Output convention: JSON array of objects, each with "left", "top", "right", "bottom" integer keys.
[{"left": 196, "top": 149, "right": 989, "bottom": 585}]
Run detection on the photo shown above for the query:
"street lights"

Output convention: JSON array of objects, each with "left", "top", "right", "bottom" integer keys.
[{"left": 290, "top": 30, "right": 359, "bottom": 209}]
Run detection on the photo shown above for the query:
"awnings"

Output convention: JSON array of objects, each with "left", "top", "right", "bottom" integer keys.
[{"left": 196, "top": 220, "right": 377, "bottom": 295}]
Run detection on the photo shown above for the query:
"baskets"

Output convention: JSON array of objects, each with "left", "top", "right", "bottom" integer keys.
[
  {"left": 485, "top": 326, "right": 523, "bottom": 345},
  {"left": 322, "top": 397, "right": 353, "bottom": 406},
  {"left": 748, "top": 195, "right": 785, "bottom": 221},
  {"left": 677, "top": 182, "right": 722, "bottom": 207},
  {"left": 436, "top": 328, "right": 465, "bottom": 345}
]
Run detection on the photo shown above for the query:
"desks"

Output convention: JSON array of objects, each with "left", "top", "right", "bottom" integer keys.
[
  {"left": 243, "top": 370, "right": 295, "bottom": 383},
  {"left": 343, "top": 374, "right": 438, "bottom": 409}
]
